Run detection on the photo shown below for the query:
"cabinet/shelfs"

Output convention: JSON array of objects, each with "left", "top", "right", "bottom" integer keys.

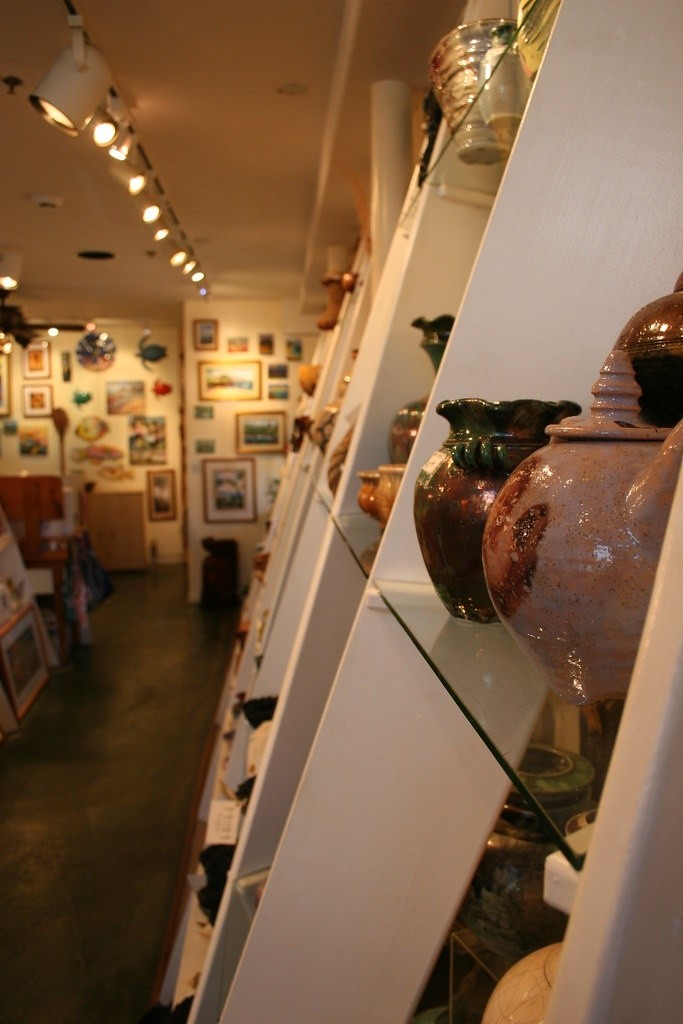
[{"left": 155, "top": 1, "right": 682, "bottom": 1024}]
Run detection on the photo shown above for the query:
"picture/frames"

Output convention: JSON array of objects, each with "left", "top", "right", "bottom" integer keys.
[
  {"left": 0, "top": 351, "right": 13, "bottom": 417},
  {"left": 193, "top": 318, "right": 219, "bottom": 350},
  {"left": 236, "top": 410, "right": 288, "bottom": 454},
  {"left": 199, "top": 359, "right": 262, "bottom": 402},
  {"left": 203, "top": 456, "right": 258, "bottom": 523},
  {"left": 147, "top": 470, "right": 177, "bottom": 522},
  {"left": 22, "top": 342, "right": 51, "bottom": 379},
  {"left": 1, "top": 603, "right": 53, "bottom": 722},
  {"left": 22, "top": 384, "right": 55, "bottom": 417}
]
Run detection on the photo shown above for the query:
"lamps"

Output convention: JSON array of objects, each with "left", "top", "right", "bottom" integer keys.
[
  {"left": 32, "top": 11, "right": 207, "bottom": 285},
  {"left": 0, "top": 252, "right": 22, "bottom": 290}
]
